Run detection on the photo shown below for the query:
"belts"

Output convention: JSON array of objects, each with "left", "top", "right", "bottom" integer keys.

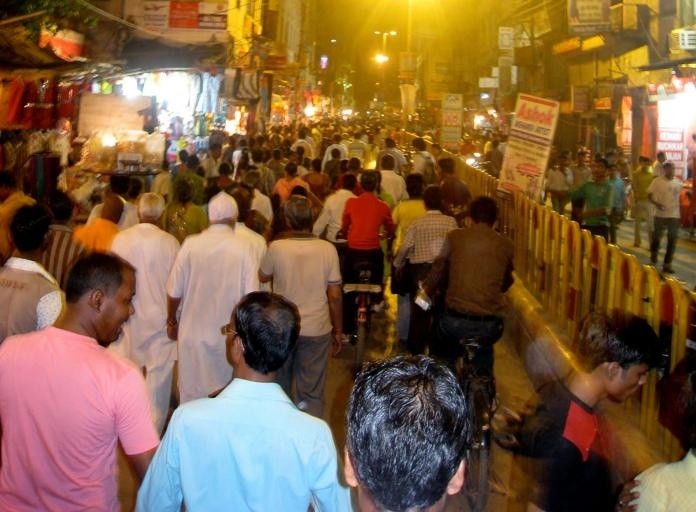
[{"left": 449, "top": 311, "right": 499, "bottom": 321}]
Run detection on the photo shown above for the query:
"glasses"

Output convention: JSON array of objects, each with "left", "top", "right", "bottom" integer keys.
[{"left": 221, "top": 324, "right": 246, "bottom": 352}]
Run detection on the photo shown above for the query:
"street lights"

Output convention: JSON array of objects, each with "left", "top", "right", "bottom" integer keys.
[{"left": 368, "top": 27, "right": 395, "bottom": 108}]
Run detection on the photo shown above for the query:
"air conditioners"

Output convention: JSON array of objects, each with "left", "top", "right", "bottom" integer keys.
[{"left": 671, "top": 29, "right": 696, "bottom": 51}]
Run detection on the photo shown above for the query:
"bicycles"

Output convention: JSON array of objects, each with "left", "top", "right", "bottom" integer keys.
[{"left": 323, "top": 272, "right": 397, "bottom": 374}]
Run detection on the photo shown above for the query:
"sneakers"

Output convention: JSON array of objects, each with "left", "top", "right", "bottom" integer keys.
[
  {"left": 370, "top": 300, "right": 385, "bottom": 313},
  {"left": 650, "top": 251, "right": 658, "bottom": 265},
  {"left": 340, "top": 334, "right": 351, "bottom": 344},
  {"left": 662, "top": 264, "right": 676, "bottom": 274}
]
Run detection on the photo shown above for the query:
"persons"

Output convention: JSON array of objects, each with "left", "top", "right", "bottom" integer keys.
[{"left": 1, "top": 110, "right": 696, "bottom": 512}]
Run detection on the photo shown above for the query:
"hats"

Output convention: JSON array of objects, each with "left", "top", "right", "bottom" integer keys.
[{"left": 208, "top": 192, "right": 238, "bottom": 222}]
